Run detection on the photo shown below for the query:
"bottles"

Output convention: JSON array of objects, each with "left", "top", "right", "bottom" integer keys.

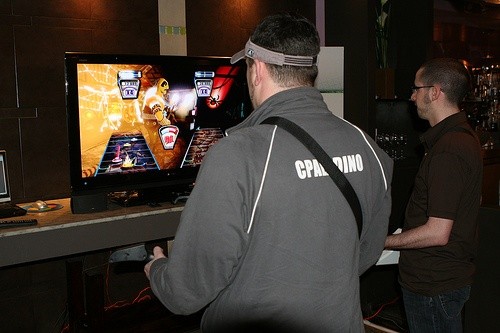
[{"left": 376, "top": 135, "right": 405, "bottom": 159}]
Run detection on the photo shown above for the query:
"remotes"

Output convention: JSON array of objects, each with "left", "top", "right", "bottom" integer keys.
[{"left": 0, "top": 219, "right": 38, "bottom": 228}]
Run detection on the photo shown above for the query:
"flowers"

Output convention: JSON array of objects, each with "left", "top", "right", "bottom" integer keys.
[{"left": 372, "top": 0, "right": 390, "bottom": 69}]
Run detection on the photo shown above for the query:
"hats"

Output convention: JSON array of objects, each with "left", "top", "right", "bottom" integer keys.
[{"left": 230, "top": 36, "right": 320, "bottom": 67}]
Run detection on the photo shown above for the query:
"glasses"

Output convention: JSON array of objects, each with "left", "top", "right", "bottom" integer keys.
[{"left": 411, "top": 85, "right": 447, "bottom": 96}]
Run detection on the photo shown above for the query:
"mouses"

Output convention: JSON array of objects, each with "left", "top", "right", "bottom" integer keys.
[{"left": 31, "top": 200, "right": 47, "bottom": 209}]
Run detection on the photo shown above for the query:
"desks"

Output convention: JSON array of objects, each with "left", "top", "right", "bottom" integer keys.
[{"left": 0, "top": 197, "right": 185, "bottom": 333}]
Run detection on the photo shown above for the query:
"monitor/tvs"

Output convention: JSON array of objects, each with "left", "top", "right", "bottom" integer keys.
[{"left": 64, "top": 51, "right": 255, "bottom": 208}]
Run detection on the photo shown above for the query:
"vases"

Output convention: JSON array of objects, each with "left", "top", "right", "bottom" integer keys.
[{"left": 374, "top": 69, "right": 395, "bottom": 99}]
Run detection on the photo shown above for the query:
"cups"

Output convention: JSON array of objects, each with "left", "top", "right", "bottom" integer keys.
[{"left": 134, "top": 88, "right": 197, "bottom": 171}]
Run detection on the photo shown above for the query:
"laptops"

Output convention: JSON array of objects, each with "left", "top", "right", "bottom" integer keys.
[{"left": 0, "top": 149, "right": 27, "bottom": 218}]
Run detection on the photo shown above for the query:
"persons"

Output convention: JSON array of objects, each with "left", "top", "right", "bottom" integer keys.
[
  {"left": 384, "top": 58, "right": 484, "bottom": 331},
  {"left": 143, "top": 15, "right": 394, "bottom": 333}
]
